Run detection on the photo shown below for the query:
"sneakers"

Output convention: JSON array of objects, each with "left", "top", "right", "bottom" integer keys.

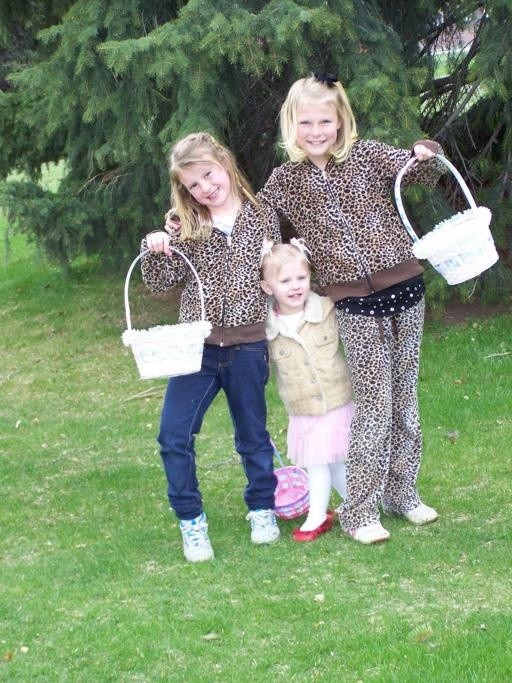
[
  {"left": 245, "top": 509, "right": 279, "bottom": 545},
  {"left": 177, "top": 516, "right": 216, "bottom": 564},
  {"left": 404, "top": 502, "right": 438, "bottom": 528},
  {"left": 346, "top": 522, "right": 390, "bottom": 542}
]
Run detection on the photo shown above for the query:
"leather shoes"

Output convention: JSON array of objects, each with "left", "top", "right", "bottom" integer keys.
[{"left": 292, "top": 511, "right": 334, "bottom": 542}]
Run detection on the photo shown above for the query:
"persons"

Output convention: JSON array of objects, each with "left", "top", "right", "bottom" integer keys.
[
  {"left": 163, "top": 71, "right": 450, "bottom": 545},
  {"left": 258, "top": 235, "right": 356, "bottom": 541},
  {"left": 138, "top": 131, "right": 284, "bottom": 561}
]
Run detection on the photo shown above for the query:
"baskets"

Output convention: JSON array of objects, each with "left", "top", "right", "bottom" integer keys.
[
  {"left": 268, "top": 462, "right": 309, "bottom": 520},
  {"left": 121, "top": 320, "right": 214, "bottom": 383},
  {"left": 408, "top": 205, "right": 500, "bottom": 287}
]
